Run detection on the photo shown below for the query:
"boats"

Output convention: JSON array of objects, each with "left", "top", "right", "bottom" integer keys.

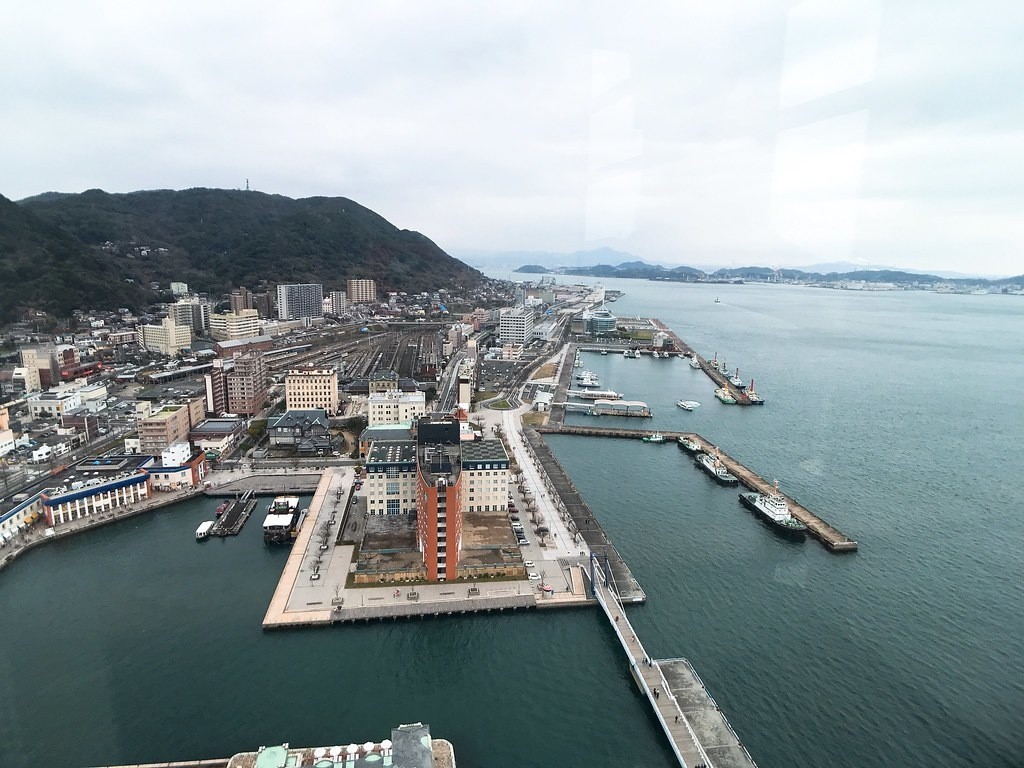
[
  {"left": 678, "top": 435, "right": 702, "bottom": 451},
  {"left": 260, "top": 493, "right": 301, "bottom": 542},
  {"left": 642, "top": 430, "right": 667, "bottom": 443},
  {"left": 573, "top": 346, "right": 766, "bottom": 405},
  {"left": 696, "top": 446, "right": 739, "bottom": 486},
  {"left": 738, "top": 480, "right": 808, "bottom": 537},
  {"left": 677, "top": 400, "right": 693, "bottom": 412},
  {"left": 195, "top": 520, "right": 215, "bottom": 539}
]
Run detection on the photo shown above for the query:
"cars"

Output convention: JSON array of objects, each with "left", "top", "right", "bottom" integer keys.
[{"left": 508, "top": 490, "right": 526, "bottom": 541}]
